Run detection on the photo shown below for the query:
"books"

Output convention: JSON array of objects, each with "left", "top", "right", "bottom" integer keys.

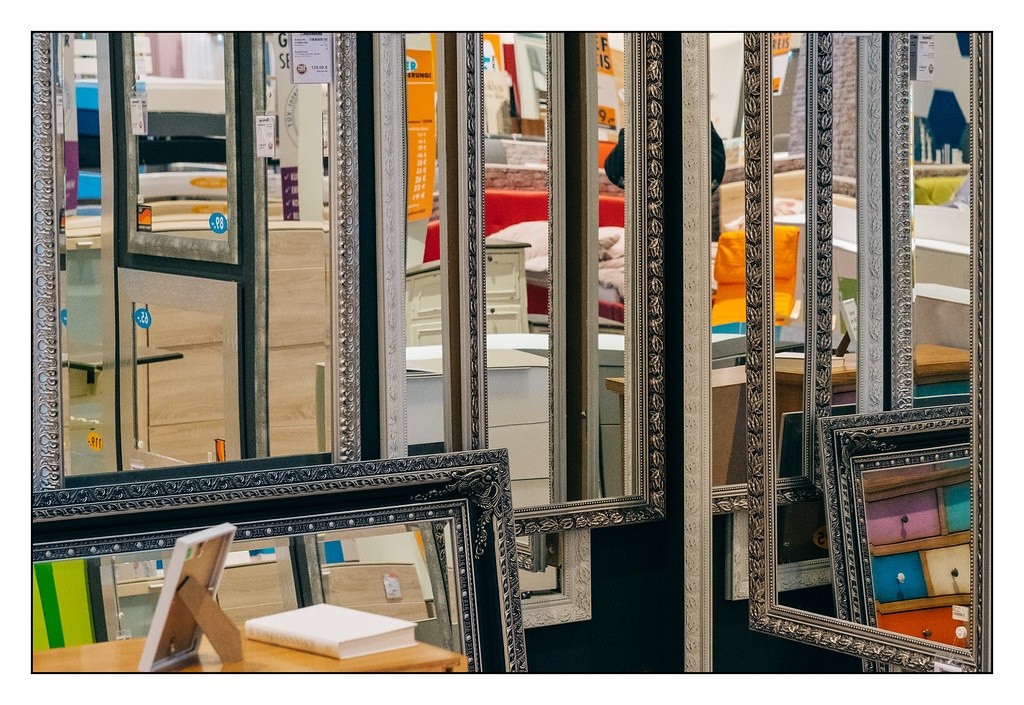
[
  {"left": 245, "top": 603, "right": 419, "bottom": 660},
  {"left": 775, "top": 352, "right": 845, "bottom": 371}
]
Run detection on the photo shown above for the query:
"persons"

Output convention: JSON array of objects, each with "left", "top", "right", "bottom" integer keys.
[{"left": 604, "top": 120, "right": 726, "bottom": 195}]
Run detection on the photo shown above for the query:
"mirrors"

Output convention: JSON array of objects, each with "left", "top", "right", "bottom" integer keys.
[{"left": 31, "top": 31, "right": 993, "bottom": 674}]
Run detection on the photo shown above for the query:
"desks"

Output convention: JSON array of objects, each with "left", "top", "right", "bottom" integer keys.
[{"left": 33, "top": 623, "right": 469, "bottom": 672}]
